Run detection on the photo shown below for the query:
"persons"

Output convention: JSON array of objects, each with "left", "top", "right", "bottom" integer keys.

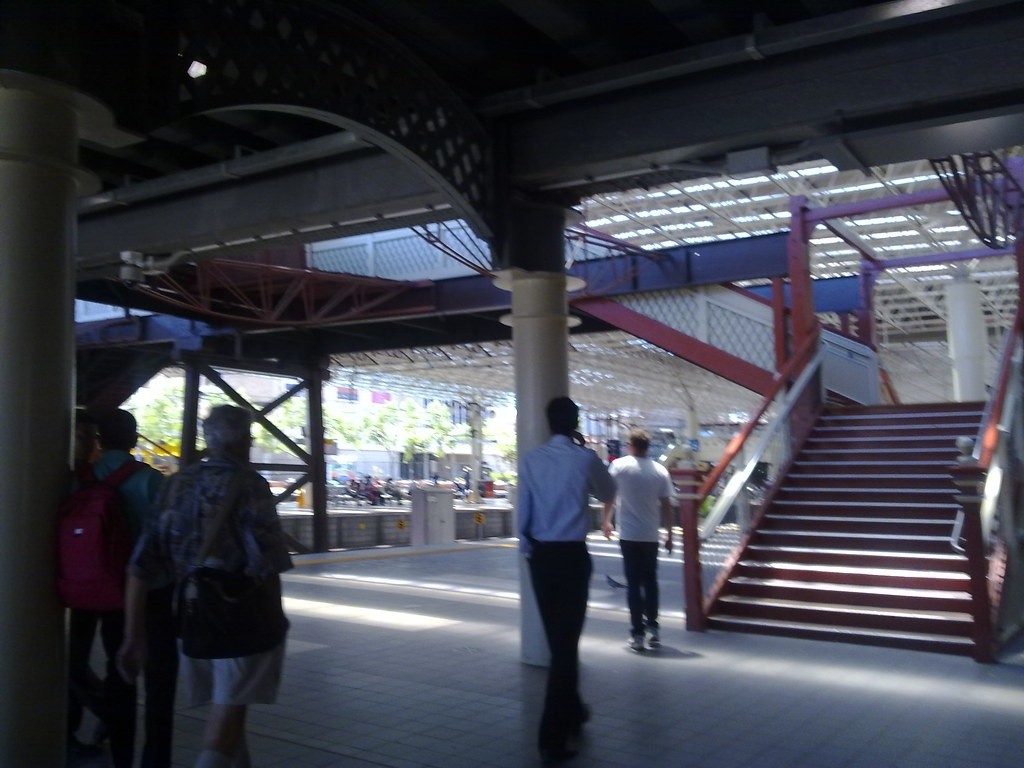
[
  {"left": 56, "top": 409, "right": 169, "bottom": 767},
  {"left": 516, "top": 396, "right": 617, "bottom": 766},
  {"left": 602, "top": 430, "right": 675, "bottom": 654},
  {"left": 113, "top": 406, "right": 294, "bottom": 767}
]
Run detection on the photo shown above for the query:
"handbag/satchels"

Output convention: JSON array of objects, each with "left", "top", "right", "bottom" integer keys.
[{"left": 180, "top": 565, "right": 289, "bottom": 658}]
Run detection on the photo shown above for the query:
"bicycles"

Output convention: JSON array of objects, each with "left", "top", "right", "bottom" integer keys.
[{"left": 339, "top": 489, "right": 409, "bottom": 508}]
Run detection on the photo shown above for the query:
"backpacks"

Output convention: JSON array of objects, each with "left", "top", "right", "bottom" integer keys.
[{"left": 56, "top": 461, "right": 155, "bottom": 613}]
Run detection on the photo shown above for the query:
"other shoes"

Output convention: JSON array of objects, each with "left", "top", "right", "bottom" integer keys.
[
  {"left": 646, "top": 633, "right": 660, "bottom": 647},
  {"left": 630, "top": 639, "right": 645, "bottom": 651},
  {"left": 563, "top": 705, "right": 589, "bottom": 726},
  {"left": 541, "top": 738, "right": 579, "bottom": 764}
]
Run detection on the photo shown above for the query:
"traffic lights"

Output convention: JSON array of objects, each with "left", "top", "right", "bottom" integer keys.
[{"left": 608, "top": 441, "right": 617, "bottom": 465}]
